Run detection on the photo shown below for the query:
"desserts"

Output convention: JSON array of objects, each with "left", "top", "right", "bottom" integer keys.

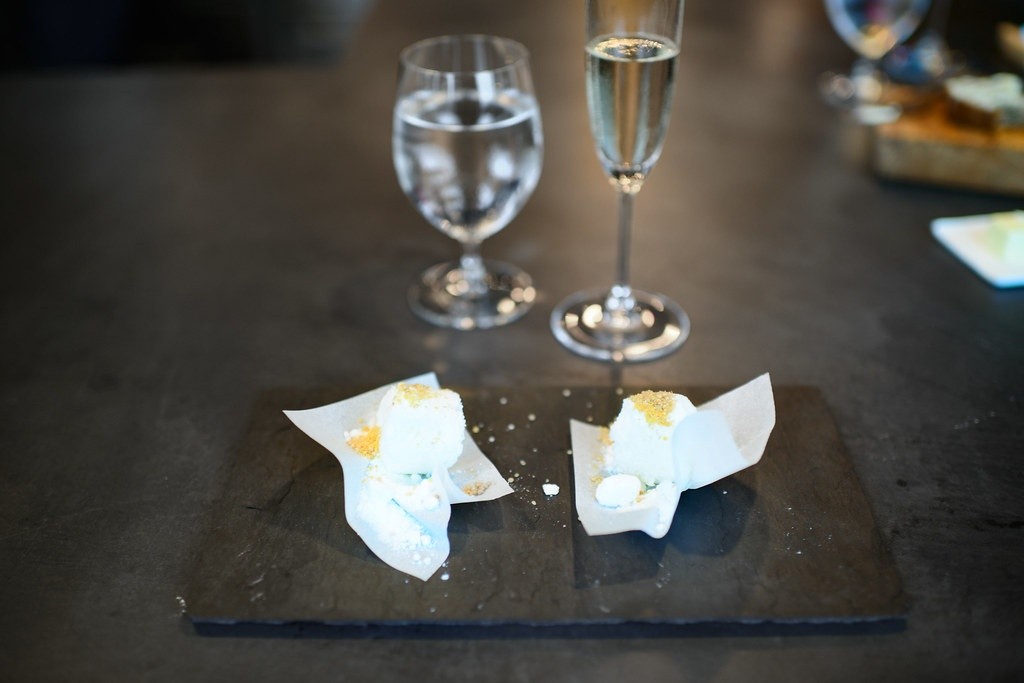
[
  {"left": 345, "top": 383, "right": 466, "bottom": 479},
  {"left": 595, "top": 389, "right": 698, "bottom": 508}
]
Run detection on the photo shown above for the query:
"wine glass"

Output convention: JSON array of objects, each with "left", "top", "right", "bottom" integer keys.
[
  {"left": 552, "top": 0, "right": 688, "bottom": 363},
  {"left": 824, "top": 0, "right": 925, "bottom": 120},
  {"left": 390, "top": 35, "right": 544, "bottom": 330}
]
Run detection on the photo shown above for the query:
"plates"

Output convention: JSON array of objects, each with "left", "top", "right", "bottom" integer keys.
[{"left": 189, "top": 388, "right": 915, "bottom": 627}]
